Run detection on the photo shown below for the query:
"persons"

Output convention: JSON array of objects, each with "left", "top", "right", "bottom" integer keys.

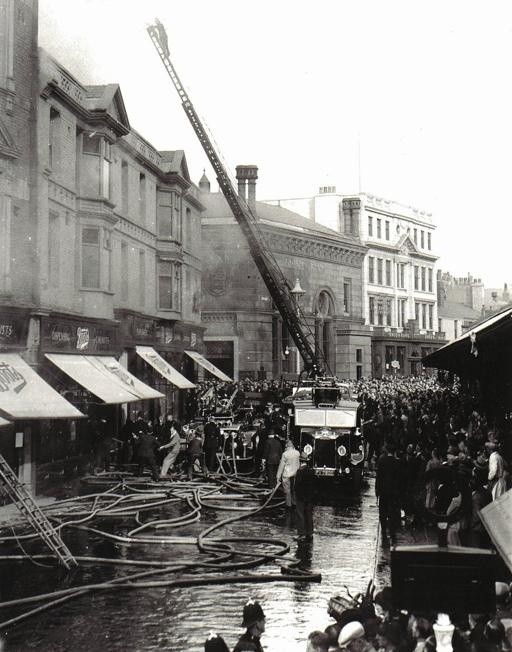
[
  {"left": 231, "top": 599, "right": 268, "bottom": 652},
  {"left": 303, "top": 575, "right": 512, "bottom": 652}
]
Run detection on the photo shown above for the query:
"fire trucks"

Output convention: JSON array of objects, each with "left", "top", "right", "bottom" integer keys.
[{"left": 148, "top": 27, "right": 369, "bottom": 507}]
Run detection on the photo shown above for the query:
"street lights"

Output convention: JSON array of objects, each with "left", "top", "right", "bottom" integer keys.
[{"left": 285, "top": 277, "right": 306, "bottom": 375}]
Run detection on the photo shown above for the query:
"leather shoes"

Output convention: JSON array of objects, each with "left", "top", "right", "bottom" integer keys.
[
  {"left": 294, "top": 535, "right": 313, "bottom": 541},
  {"left": 279, "top": 505, "right": 296, "bottom": 510}
]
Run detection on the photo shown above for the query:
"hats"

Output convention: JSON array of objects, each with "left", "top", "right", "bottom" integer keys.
[
  {"left": 298, "top": 452, "right": 310, "bottom": 461},
  {"left": 267, "top": 429, "right": 276, "bottom": 435},
  {"left": 473, "top": 456, "right": 489, "bottom": 469},
  {"left": 240, "top": 597, "right": 266, "bottom": 627},
  {"left": 338, "top": 609, "right": 365, "bottom": 648},
  {"left": 375, "top": 586, "right": 395, "bottom": 611}
]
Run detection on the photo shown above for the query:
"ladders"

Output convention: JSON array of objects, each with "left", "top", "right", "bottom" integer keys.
[{"left": 0, "top": 454, "right": 79, "bottom": 571}]
